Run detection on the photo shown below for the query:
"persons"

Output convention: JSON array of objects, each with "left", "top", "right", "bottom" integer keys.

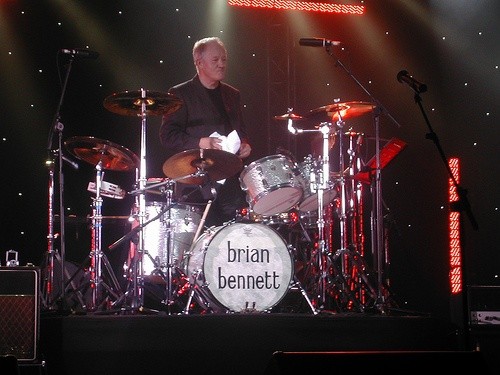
[
  {"left": 159, "top": 38, "right": 252, "bottom": 228},
  {"left": 304, "top": 123, "right": 343, "bottom": 173}
]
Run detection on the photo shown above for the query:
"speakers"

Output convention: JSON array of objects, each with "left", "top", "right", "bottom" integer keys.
[{"left": 0, "top": 265, "right": 42, "bottom": 362}]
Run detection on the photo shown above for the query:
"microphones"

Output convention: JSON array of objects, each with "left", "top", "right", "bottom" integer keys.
[
  {"left": 63, "top": 48, "right": 100, "bottom": 59},
  {"left": 396, "top": 69, "right": 427, "bottom": 92},
  {"left": 298, "top": 37, "right": 340, "bottom": 47}
]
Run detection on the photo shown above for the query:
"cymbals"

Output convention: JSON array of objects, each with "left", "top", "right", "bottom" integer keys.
[
  {"left": 103, "top": 90, "right": 181, "bottom": 117},
  {"left": 303, "top": 100, "right": 378, "bottom": 121},
  {"left": 162, "top": 149, "right": 243, "bottom": 184},
  {"left": 87, "top": 180, "right": 125, "bottom": 199},
  {"left": 273, "top": 114, "right": 303, "bottom": 120},
  {"left": 64, "top": 136, "right": 140, "bottom": 170}
]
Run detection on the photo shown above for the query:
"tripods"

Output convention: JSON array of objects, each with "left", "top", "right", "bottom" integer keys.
[
  {"left": 42, "top": 64, "right": 216, "bottom": 315},
  {"left": 278, "top": 47, "right": 411, "bottom": 315}
]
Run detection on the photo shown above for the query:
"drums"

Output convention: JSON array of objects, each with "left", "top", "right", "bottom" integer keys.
[
  {"left": 294, "top": 158, "right": 339, "bottom": 212},
  {"left": 124, "top": 201, "right": 203, "bottom": 285},
  {"left": 184, "top": 220, "right": 294, "bottom": 315},
  {"left": 240, "top": 154, "right": 304, "bottom": 216},
  {"left": 236, "top": 208, "right": 295, "bottom": 226}
]
